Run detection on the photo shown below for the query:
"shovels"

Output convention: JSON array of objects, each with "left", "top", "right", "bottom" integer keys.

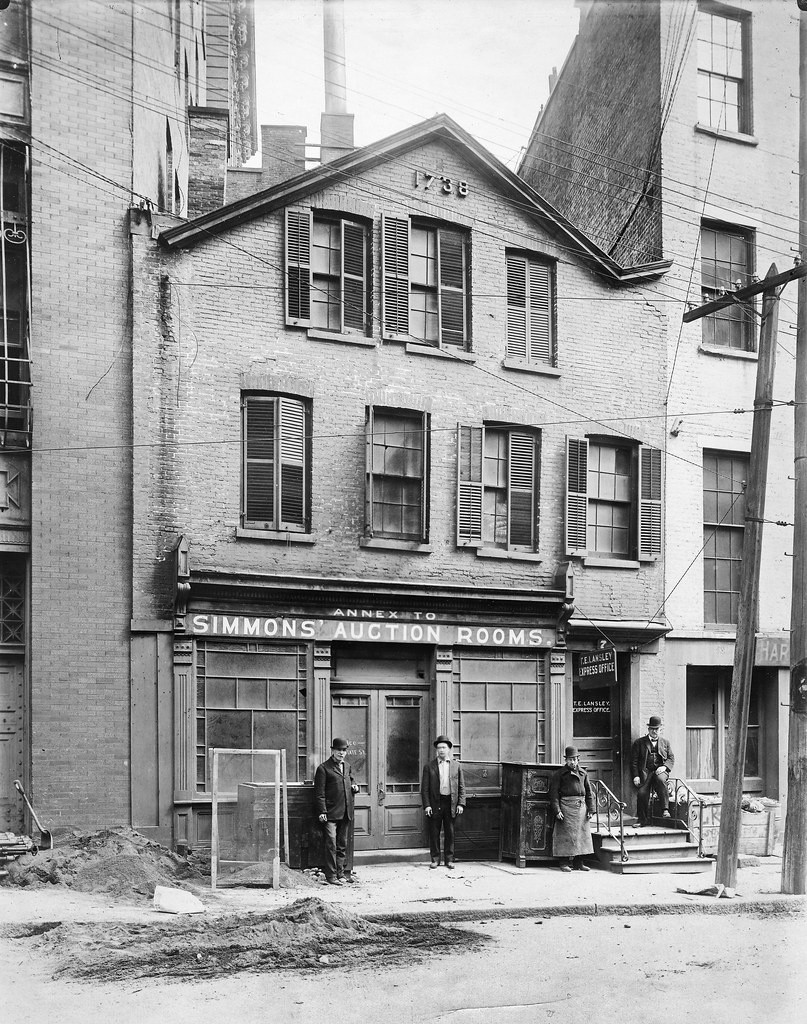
[{"left": 13, "top": 779, "right": 54, "bottom": 849}]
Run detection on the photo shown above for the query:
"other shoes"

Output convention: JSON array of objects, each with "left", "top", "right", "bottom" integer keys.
[
  {"left": 430, "top": 861, "right": 440, "bottom": 869},
  {"left": 445, "top": 860, "right": 455, "bottom": 869},
  {"left": 559, "top": 862, "right": 572, "bottom": 872},
  {"left": 632, "top": 820, "right": 646, "bottom": 828},
  {"left": 339, "top": 874, "right": 349, "bottom": 882},
  {"left": 573, "top": 863, "right": 591, "bottom": 872},
  {"left": 326, "top": 876, "right": 343, "bottom": 885},
  {"left": 660, "top": 808, "right": 672, "bottom": 818}
]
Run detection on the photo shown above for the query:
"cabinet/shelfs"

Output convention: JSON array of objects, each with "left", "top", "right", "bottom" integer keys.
[
  {"left": 238, "top": 781, "right": 355, "bottom": 873},
  {"left": 500, "top": 762, "right": 589, "bottom": 867}
]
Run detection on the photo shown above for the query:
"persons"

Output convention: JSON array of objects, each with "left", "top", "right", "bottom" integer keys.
[
  {"left": 421, "top": 735, "right": 466, "bottom": 869},
  {"left": 313, "top": 738, "right": 359, "bottom": 885},
  {"left": 630, "top": 716, "right": 675, "bottom": 828},
  {"left": 549, "top": 746, "right": 596, "bottom": 872}
]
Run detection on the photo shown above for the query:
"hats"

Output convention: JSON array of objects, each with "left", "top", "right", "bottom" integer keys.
[
  {"left": 333, "top": 738, "right": 348, "bottom": 749},
  {"left": 433, "top": 735, "right": 455, "bottom": 750},
  {"left": 646, "top": 716, "right": 662, "bottom": 729},
  {"left": 563, "top": 745, "right": 581, "bottom": 759}
]
